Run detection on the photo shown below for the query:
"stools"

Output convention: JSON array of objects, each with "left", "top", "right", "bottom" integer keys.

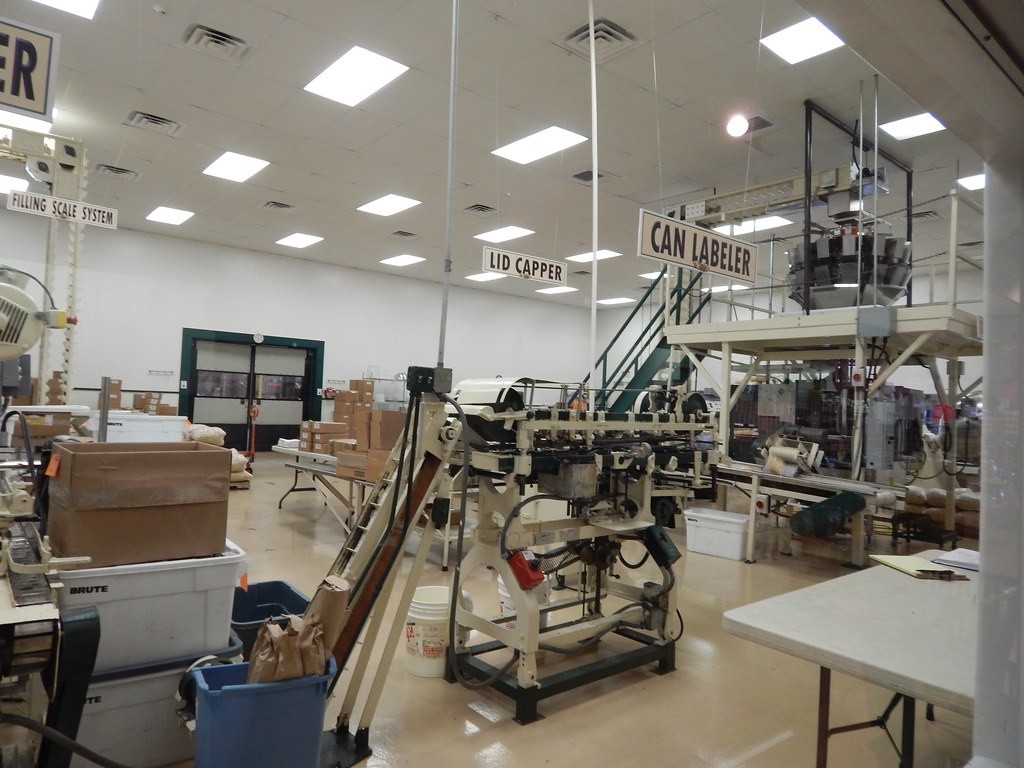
[{"left": 891, "top": 511, "right": 958, "bottom": 553}]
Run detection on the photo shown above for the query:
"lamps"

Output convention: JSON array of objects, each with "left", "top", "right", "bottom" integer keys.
[
  {"left": 849, "top": 139, "right": 889, "bottom": 197},
  {"left": 249, "top": 333, "right": 264, "bottom": 347},
  {"left": 751, "top": 375, "right": 769, "bottom": 384}
]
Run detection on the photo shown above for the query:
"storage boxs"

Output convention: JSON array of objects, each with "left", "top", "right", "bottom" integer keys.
[
  {"left": 683, "top": 506, "right": 749, "bottom": 561},
  {"left": 8, "top": 365, "right": 410, "bottom": 768}
]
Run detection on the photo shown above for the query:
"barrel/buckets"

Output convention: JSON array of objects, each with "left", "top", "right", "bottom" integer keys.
[
  {"left": 497, "top": 571, "right": 553, "bottom": 630},
  {"left": 402, "top": 586, "right": 473, "bottom": 678}
]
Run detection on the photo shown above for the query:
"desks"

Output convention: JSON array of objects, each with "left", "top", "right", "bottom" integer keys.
[
  {"left": 272, "top": 445, "right": 339, "bottom": 509},
  {"left": 286, "top": 461, "right": 378, "bottom": 539},
  {"left": 723, "top": 549, "right": 981, "bottom": 768}
]
